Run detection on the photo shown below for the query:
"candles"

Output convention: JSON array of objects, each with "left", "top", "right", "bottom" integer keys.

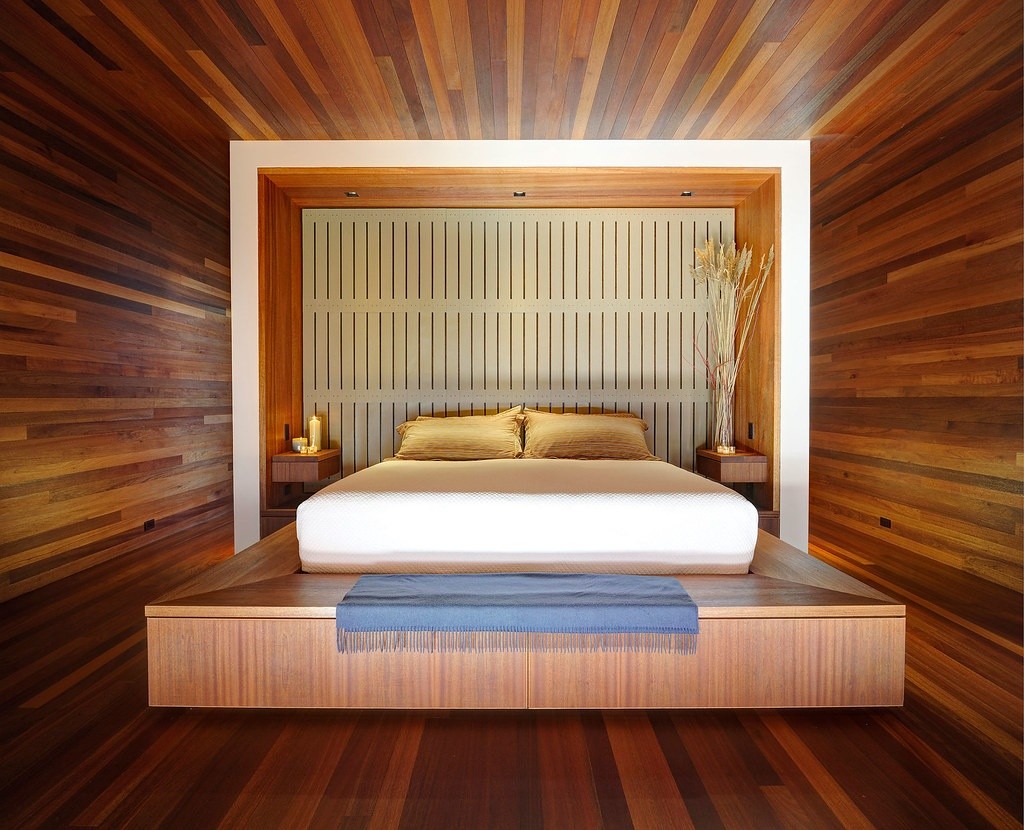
[
  {"left": 292, "top": 437, "right": 308, "bottom": 453},
  {"left": 730, "top": 446, "right": 736, "bottom": 454},
  {"left": 312, "top": 446, "right": 317, "bottom": 452},
  {"left": 300, "top": 445, "right": 308, "bottom": 454},
  {"left": 723, "top": 447, "right": 730, "bottom": 454},
  {"left": 308, "top": 415, "right": 323, "bottom": 451},
  {"left": 717, "top": 445, "right": 723, "bottom": 454},
  {"left": 307, "top": 447, "right": 314, "bottom": 453}
]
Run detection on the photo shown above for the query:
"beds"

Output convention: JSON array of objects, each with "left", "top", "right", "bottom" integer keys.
[{"left": 143, "top": 456, "right": 908, "bottom": 710}]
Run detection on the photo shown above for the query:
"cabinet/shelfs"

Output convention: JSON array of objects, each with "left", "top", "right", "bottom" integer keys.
[{"left": 260, "top": 448, "right": 340, "bottom": 540}]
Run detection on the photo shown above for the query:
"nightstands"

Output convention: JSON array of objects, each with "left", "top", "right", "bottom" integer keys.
[{"left": 696, "top": 449, "right": 779, "bottom": 538}]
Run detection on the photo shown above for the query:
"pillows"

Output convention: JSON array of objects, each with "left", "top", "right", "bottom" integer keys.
[
  {"left": 522, "top": 406, "right": 662, "bottom": 461},
  {"left": 394, "top": 404, "right": 525, "bottom": 461}
]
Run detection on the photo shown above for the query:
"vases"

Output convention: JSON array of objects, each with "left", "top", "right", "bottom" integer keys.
[{"left": 712, "top": 389, "right": 735, "bottom": 451}]
